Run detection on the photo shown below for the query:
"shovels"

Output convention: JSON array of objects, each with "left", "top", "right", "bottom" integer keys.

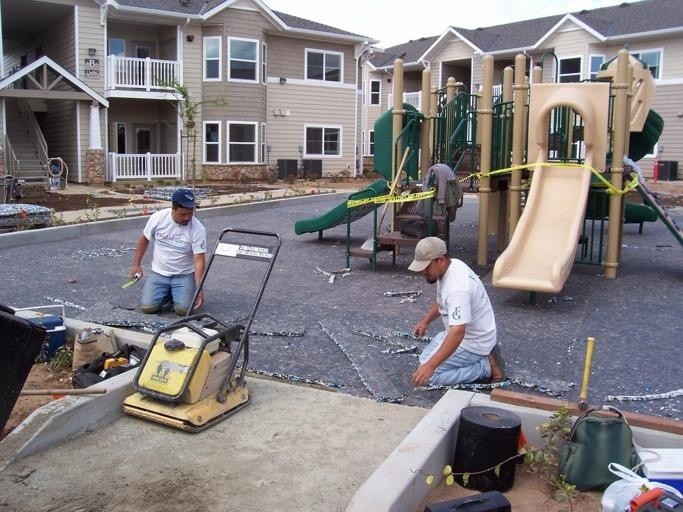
[{"left": 360, "top": 146, "right": 410, "bottom": 250}]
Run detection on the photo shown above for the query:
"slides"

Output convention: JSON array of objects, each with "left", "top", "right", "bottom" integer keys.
[
  {"left": 293, "top": 177, "right": 393, "bottom": 237},
  {"left": 492, "top": 150, "right": 594, "bottom": 295},
  {"left": 550, "top": 105, "right": 664, "bottom": 223}
]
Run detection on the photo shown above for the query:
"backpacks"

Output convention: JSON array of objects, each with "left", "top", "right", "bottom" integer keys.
[{"left": 556, "top": 403, "right": 634, "bottom": 491}]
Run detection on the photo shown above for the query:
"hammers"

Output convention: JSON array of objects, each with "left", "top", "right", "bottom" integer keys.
[{"left": 577, "top": 336, "right": 595, "bottom": 410}]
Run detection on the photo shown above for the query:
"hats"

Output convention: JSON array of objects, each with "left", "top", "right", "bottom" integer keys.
[
  {"left": 407, "top": 236, "right": 447, "bottom": 273},
  {"left": 171, "top": 187, "right": 200, "bottom": 208}
]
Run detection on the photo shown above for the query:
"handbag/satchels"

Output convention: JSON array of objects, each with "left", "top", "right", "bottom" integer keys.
[
  {"left": 70, "top": 344, "right": 147, "bottom": 388},
  {"left": 71, "top": 330, "right": 119, "bottom": 372}
]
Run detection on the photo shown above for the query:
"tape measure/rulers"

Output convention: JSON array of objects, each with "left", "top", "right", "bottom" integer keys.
[{"left": 120, "top": 271, "right": 142, "bottom": 289}]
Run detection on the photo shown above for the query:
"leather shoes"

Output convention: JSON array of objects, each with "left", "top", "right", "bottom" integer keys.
[{"left": 488, "top": 349, "right": 506, "bottom": 383}]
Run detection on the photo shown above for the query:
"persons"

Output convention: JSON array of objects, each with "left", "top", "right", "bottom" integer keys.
[
  {"left": 407, "top": 236, "right": 507, "bottom": 390},
  {"left": 127, "top": 188, "right": 207, "bottom": 317}
]
